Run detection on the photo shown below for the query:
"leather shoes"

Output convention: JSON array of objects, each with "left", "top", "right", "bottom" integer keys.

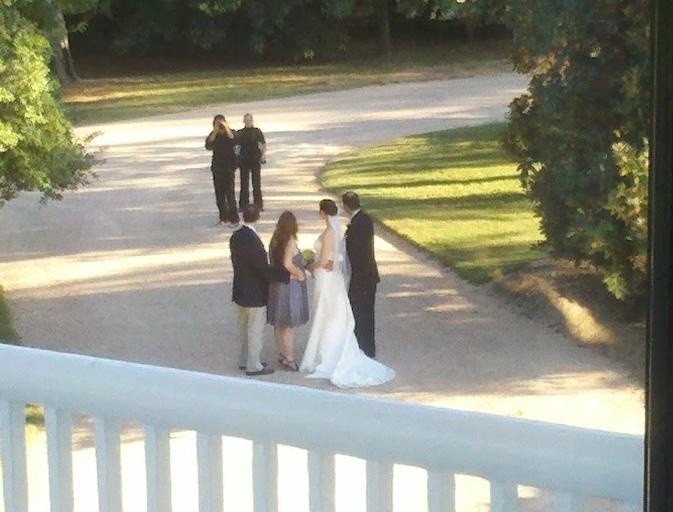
[{"left": 238, "top": 362, "right": 276, "bottom": 376}]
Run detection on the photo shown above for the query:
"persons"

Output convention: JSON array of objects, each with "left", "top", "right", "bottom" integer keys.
[
  {"left": 268, "top": 210, "right": 310, "bottom": 372},
  {"left": 205, "top": 114, "right": 242, "bottom": 228},
  {"left": 228, "top": 203, "right": 301, "bottom": 377},
  {"left": 342, "top": 191, "right": 381, "bottom": 359},
  {"left": 303, "top": 199, "right": 346, "bottom": 387},
  {"left": 235, "top": 113, "right": 267, "bottom": 212}
]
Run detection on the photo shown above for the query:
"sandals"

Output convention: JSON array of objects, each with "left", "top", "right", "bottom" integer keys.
[{"left": 276, "top": 353, "right": 302, "bottom": 372}]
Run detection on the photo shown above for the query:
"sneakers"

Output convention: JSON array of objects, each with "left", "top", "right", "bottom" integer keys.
[{"left": 214, "top": 206, "right": 267, "bottom": 229}]
